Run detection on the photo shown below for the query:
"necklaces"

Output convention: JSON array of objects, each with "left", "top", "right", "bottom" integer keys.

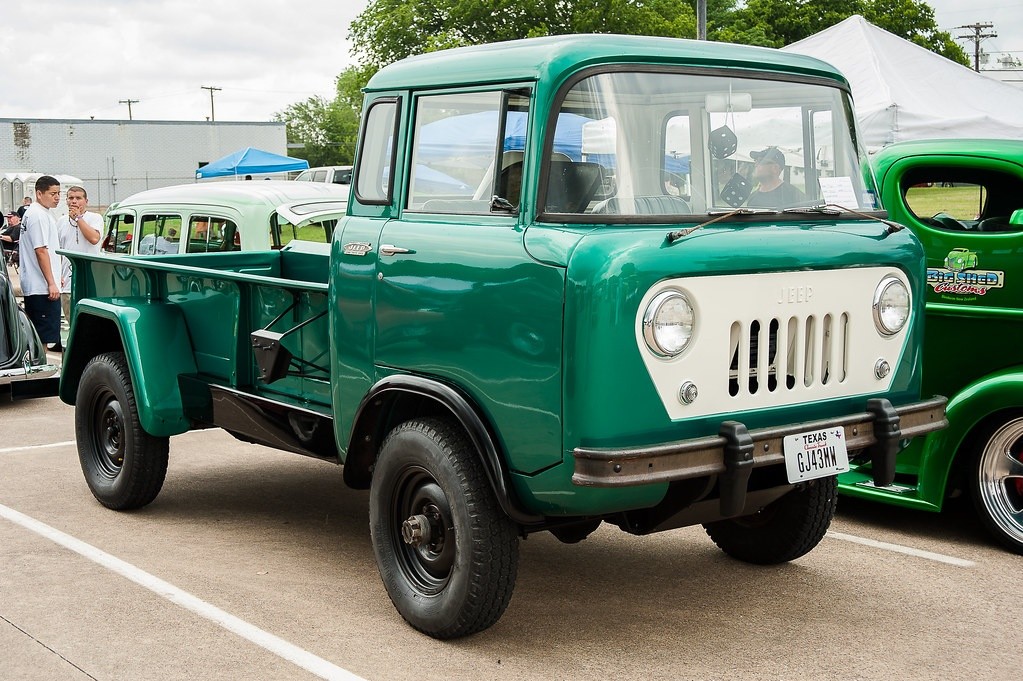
[{"left": 69, "top": 211, "right": 87, "bottom": 227}]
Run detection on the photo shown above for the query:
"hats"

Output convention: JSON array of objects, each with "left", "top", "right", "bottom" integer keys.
[
  {"left": 750, "top": 147, "right": 785, "bottom": 170},
  {"left": 3, "top": 210, "right": 20, "bottom": 219}
]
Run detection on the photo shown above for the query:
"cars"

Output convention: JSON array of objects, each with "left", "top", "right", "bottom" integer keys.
[{"left": 0, "top": 235, "right": 63, "bottom": 386}]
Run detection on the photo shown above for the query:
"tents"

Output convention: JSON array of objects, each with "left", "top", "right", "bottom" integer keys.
[
  {"left": 386, "top": 110, "right": 692, "bottom": 174},
  {"left": 195, "top": 147, "right": 311, "bottom": 181},
  {"left": 581, "top": 14, "right": 1023, "bottom": 162}
]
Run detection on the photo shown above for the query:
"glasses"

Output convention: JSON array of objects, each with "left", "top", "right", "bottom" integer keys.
[{"left": 753, "top": 159, "right": 775, "bottom": 166}]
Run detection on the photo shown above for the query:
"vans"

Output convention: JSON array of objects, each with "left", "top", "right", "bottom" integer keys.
[
  {"left": 98, "top": 177, "right": 349, "bottom": 265},
  {"left": 290, "top": 164, "right": 356, "bottom": 223}
]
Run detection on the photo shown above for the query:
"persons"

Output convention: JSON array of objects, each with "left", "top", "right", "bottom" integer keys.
[
  {"left": 245, "top": 175, "right": 252, "bottom": 180},
  {"left": 0, "top": 211, "right": 21, "bottom": 255},
  {"left": 747, "top": 148, "right": 807, "bottom": 207},
  {"left": 17, "top": 197, "right": 32, "bottom": 218},
  {"left": 20, "top": 176, "right": 64, "bottom": 352},
  {"left": 195, "top": 221, "right": 226, "bottom": 243},
  {"left": 56, "top": 186, "right": 104, "bottom": 324}
]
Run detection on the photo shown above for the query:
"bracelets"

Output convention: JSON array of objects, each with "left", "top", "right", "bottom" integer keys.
[{"left": 74, "top": 215, "right": 83, "bottom": 221}]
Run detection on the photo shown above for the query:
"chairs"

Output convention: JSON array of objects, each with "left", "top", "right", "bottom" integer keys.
[
  {"left": 977, "top": 215, "right": 1011, "bottom": 233},
  {"left": 591, "top": 194, "right": 691, "bottom": 214},
  {"left": 422, "top": 150, "right": 572, "bottom": 217}
]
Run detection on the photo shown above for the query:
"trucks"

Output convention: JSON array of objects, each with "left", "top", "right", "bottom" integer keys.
[
  {"left": 53, "top": 31, "right": 953, "bottom": 640},
  {"left": 828, "top": 137, "right": 1023, "bottom": 557}
]
